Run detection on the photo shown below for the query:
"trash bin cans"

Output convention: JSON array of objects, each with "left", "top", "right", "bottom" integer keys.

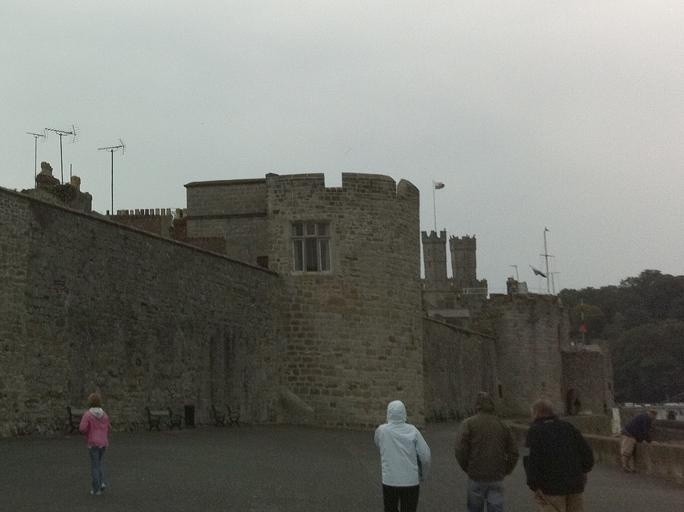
[{"left": 184, "top": 404, "right": 195, "bottom": 428}]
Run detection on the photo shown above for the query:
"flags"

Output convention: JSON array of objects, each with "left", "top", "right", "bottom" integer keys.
[{"left": 434, "top": 181, "right": 446, "bottom": 190}]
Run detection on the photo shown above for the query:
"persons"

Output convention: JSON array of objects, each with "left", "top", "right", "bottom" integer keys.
[
  {"left": 522, "top": 399, "right": 595, "bottom": 511},
  {"left": 573, "top": 397, "right": 581, "bottom": 414},
  {"left": 619, "top": 409, "right": 658, "bottom": 473},
  {"left": 453, "top": 391, "right": 519, "bottom": 511},
  {"left": 79, "top": 393, "right": 112, "bottom": 495},
  {"left": 373, "top": 400, "right": 430, "bottom": 512}
]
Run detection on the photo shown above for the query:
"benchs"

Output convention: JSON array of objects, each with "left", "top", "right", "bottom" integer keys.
[
  {"left": 145, "top": 406, "right": 183, "bottom": 432},
  {"left": 67, "top": 406, "right": 87, "bottom": 434},
  {"left": 212, "top": 402, "right": 240, "bottom": 428}
]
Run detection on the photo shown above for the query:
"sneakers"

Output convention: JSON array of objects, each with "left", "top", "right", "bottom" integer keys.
[{"left": 91, "top": 482, "right": 107, "bottom": 496}]
